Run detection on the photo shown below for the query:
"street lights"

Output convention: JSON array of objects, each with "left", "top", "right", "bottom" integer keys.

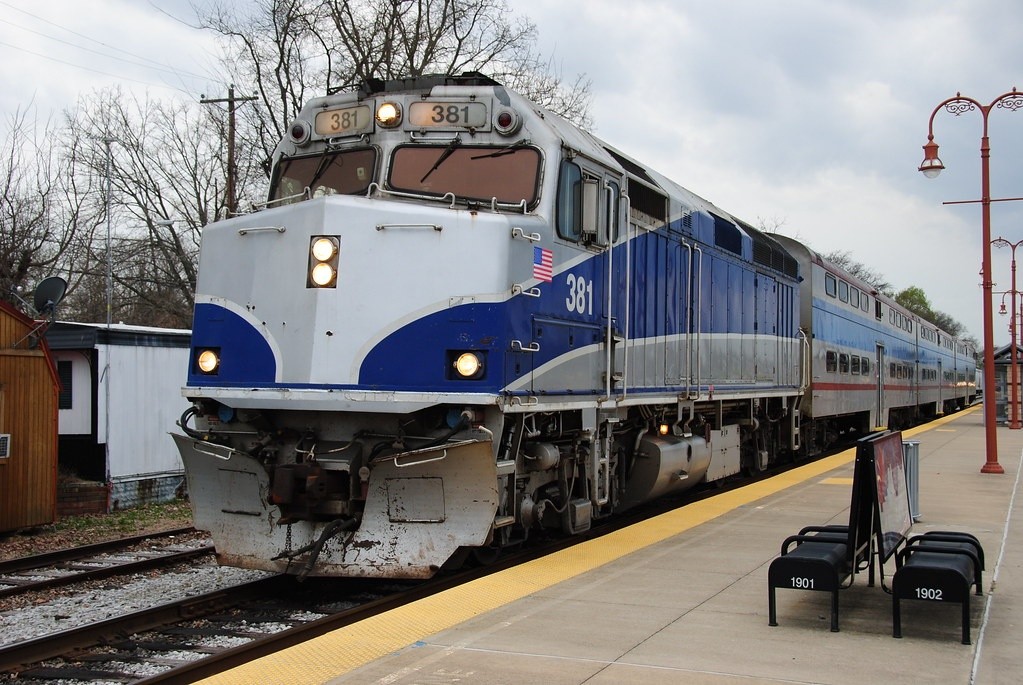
[
  {"left": 918, "top": 86, "right": 1023, "bottom": 474},
  {"left": 979, "top": 237, "right": 1023, "bottom": 429}
]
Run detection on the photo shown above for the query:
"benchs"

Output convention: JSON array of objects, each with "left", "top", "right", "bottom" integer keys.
[
  {"left": 892, "top": 531, "right": 984, "bottom": 645},
  {"left": 768, "top": 524, "right": 875, "bottom": 631}
]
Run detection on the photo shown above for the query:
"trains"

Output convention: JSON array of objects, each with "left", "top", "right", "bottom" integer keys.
[{"left": 168, "top": 70, "right": 986, "bottom": 581}]
